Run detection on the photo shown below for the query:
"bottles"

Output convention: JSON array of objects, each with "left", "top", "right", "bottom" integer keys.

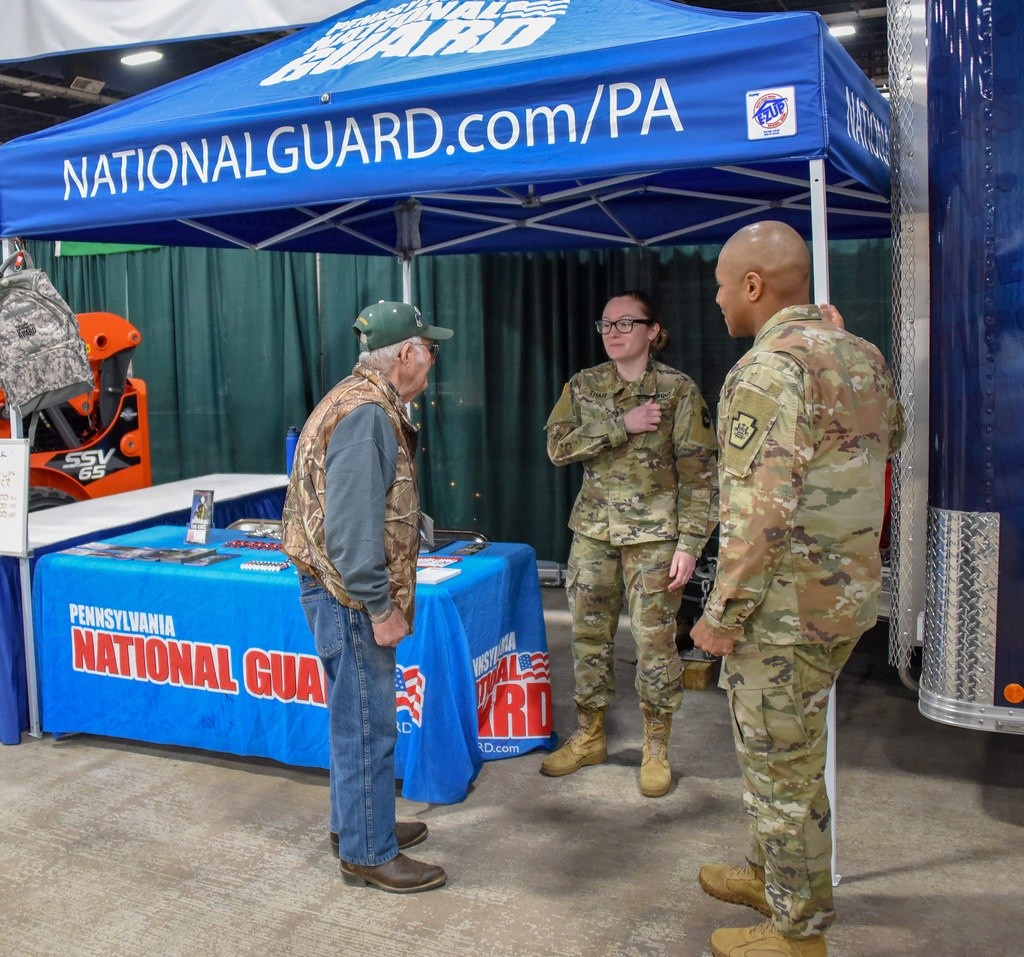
[{"left": 286, "top": 426, "right": 301, "bottom": 478}]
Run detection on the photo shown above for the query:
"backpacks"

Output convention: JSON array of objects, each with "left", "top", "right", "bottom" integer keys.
[{"left": 0, "top": 246, "right": 94, "bottom": 419}]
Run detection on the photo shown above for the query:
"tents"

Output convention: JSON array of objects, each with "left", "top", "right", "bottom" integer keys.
[{"left": 0, "top": 1, "right": 898, "bottom": 886}]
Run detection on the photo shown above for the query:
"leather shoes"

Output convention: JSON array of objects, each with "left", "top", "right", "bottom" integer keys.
[
  {"left": 340, "top": 851, "right": 447, "bottom": 894},
  {"left": 329, "top": 820, "right": 428, "bottom": 858}
]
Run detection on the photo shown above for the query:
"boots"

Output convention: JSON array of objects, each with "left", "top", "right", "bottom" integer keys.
[
  {"left": 639, "top": 702, "right": 671, "bottom": 796},
  {"left": 541, "top": 707, "right": 608, "bottom": 776}
]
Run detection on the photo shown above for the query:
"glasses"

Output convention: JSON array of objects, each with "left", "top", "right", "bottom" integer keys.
[
  {"left": 595, "top": 319, "right": 657, "bottom": 334},
  {"left": 398, "top": 343, "right": 440, "bottom": 360}
]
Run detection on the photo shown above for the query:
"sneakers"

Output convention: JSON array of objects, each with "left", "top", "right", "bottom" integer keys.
[
  {"left": 709, "top": 919, "right": 827, "bottom": 957},
  {"left": 699, "top": 855, "right": 773, "bottom": 917}
]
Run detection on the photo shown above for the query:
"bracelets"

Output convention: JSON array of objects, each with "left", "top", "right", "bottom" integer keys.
[{"left": 368, "top": 602, "right": 396, "bottom": 623}]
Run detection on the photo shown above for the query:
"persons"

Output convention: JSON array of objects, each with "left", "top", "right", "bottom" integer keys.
[
  {"left": 279, "top": 299, "right": 457, "bottom": 896},
  {"left": 540, "top": 289, "right": 721, "bottom": 799},
  {"left": 689, "top": 219, "right": 909, "bottom": 955},
  {"left": 194, "top": 503, "right": 207, "bottom": 518}
]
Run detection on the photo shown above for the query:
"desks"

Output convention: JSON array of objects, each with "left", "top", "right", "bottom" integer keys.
[
  {"left": 32, "top": 526, "right": 558, "bottom": 805},
  {"left": 0, "top": 474, "right": 291, "bottom": 746}
]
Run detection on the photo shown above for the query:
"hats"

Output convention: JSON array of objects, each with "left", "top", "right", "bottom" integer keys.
[{"left": 351, "top": 299, "right": 454, "bottom": 353}]
